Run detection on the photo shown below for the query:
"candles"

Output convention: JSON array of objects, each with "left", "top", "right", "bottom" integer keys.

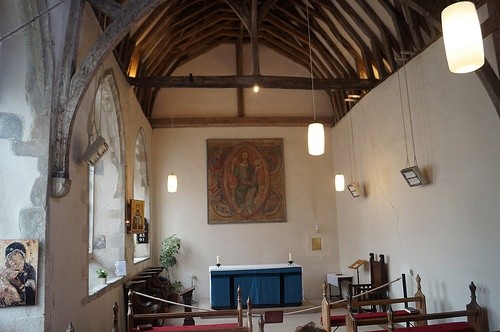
[
  {"left": 217, "top": 256, "right": 220, "bottom": 263},
  {"left": 289, "top": 253, "right": 292, "bottom": 261}
]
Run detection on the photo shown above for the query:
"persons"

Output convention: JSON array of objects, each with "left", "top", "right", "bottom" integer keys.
[{"left": 132, "top": 204, "right": 143, "bottom": 229}]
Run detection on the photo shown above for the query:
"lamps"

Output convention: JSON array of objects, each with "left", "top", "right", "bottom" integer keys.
[
  {"left": 82, "top": 15, "right": 109, "bottom": 167},
  {"left": 441, "top": 1, "right": 485, "bottom": 74},
  {"left": 394, "top": 51, "right": 428, "bottom": 187},
  {"left": 307, "top": 0, "right": 325, "bottom": 156},
  {"left": 335, "top": 148, "right": 344, "bottom": 191},
  {"left": 344, "top": 90, "right": 361, "bottom": 199},
  {"left": 167, "top": 87, "right": 177, "bottom": 193}
]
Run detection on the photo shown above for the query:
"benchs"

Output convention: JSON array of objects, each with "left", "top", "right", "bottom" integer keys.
[
  {"left": 322, "top": 275, "right": 427, "bottom": 332},
  {"left": 66, "top": 285, "right": 265, "bottom": 332},
  {"left": 346, "top": 281, "right": 484, "bottom": 332}
]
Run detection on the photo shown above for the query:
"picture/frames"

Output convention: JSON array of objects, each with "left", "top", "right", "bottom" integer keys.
[{"left": 130, "top": 200, "right": 145, "bottom": 230}]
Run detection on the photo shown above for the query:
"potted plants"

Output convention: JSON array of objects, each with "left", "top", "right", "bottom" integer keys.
[
  {"left": 160, "top": 234, "right": 181, "bottom": 303},
  {"left": 96, "top": 269, "right": 108, "bottom": 284}
]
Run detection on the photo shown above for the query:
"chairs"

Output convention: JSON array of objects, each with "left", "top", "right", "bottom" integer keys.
[
  {"left": 122, "top": 266, "right": 169, "bottom": 327},
  {"left": 349, "top": 253, "right": 387, "bottom": 314}
]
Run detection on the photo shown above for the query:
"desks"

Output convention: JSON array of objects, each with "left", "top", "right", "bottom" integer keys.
[
  {"left": 209, "top": 264, "right": 304, "bottom": 310},
  {"left": 326, "top": 273, "right": 354, "bottom": 303}
]
